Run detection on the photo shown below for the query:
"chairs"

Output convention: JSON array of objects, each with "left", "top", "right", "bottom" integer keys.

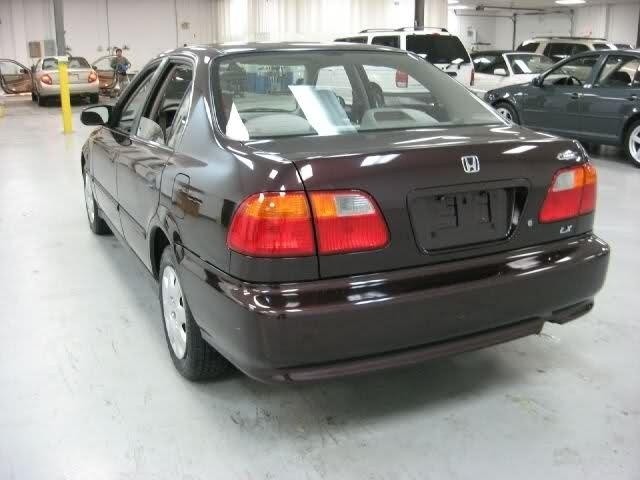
[
  {"left": 241, "top": 87, "right": 338, "bottom": 137},
  {"left": 599, "top": 71, "right": 639, "bottom": 87}
]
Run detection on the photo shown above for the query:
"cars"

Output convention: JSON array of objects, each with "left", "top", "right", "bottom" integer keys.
[
  {"left": 469, "top": 48, "right": 577, "bottom": 100},
  {"left": 81, "top": 42, "right": 611, "bottom": 385},
  {"left": 484, "top": 49, "right": 640, "bottom": 169},
  {"left": 0, "top": 56, "right": 118, "bottom": 106}
]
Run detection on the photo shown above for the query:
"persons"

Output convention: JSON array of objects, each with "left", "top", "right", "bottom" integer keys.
[{"left": 110, "top": 49, "right": 131, "bottom": 95}]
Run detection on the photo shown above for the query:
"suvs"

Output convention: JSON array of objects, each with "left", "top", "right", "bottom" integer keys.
[
  {"left": 513, "top": 36, "right": 640, "bottom": 83},
  {"left": 291, "top": 27, "right": 474, "bottom": 109}
]
